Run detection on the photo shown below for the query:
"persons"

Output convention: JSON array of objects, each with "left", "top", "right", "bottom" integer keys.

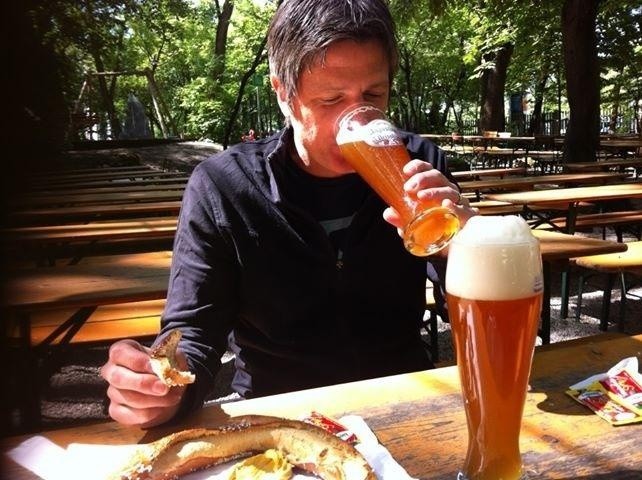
[{"left": 100, "top": 0, "right": 480, "bottom": 430}]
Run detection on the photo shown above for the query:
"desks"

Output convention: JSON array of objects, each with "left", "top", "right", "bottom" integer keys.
[{"left": 1, "top": 110, "right": 642, "bottom": 480}]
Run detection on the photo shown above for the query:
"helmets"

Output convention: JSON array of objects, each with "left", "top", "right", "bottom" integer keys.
[{"left": 51, "top": 415, "right": 418, "bottom": 479}]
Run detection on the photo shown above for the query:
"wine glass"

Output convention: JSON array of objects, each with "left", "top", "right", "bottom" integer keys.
[{"left": 457, "top": 194, "right": 463, "bottom": 206}]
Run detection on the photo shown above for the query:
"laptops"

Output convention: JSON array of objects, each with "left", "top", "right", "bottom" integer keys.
[
  {"left": 120, "top": 415, "right": 376, "bottom": 480},
  {"left": 150, "top": 329, "right": 195, "bottom": 386}
]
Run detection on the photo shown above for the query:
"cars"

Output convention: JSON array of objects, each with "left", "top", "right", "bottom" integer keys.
[
  {"left": 334, "top": 101, "right": 459, "bottom": 256},
  {"left": 445, "top": 235, "right": 544, "bottom": 479}
]
